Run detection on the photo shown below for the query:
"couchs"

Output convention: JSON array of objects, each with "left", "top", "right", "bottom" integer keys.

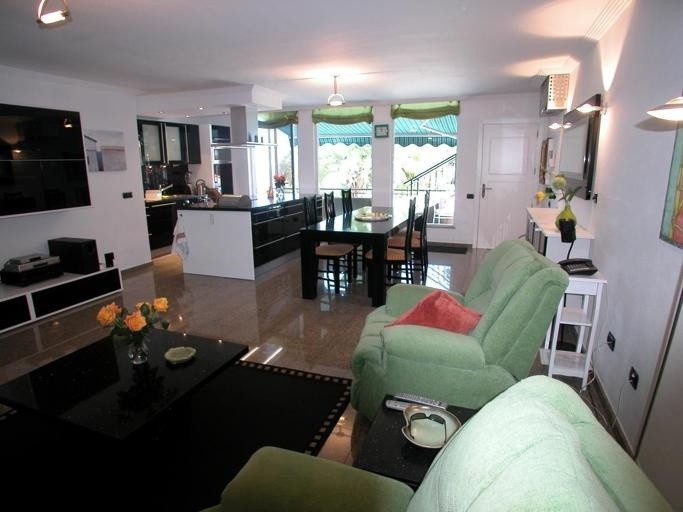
[
  {"left": 220, "top": 375, "right": 674, "bottom": 512},
  {"left": 350, "top": 238, "right": 569, "bottom": 423}
]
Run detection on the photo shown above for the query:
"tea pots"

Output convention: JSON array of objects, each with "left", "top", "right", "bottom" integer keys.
[{"left": 192, "top": 179, "right": 208, "bottom": 195}]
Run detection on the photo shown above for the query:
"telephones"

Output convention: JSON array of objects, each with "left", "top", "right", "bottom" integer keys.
[{"left": 557, "top": 258, "right": 598, "bottom": 275}]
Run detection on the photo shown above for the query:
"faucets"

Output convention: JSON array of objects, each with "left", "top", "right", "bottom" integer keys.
[{"left": 159, "top": 184, "right": 173, "bottom": 192}]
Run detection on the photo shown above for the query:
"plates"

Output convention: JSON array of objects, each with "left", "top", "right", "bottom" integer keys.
[
  {"left": 401, "top": 404, "right": 463, "bottom": 449},
  {"left": 355, "top": 212, "right": 393, "bottom": 221}
]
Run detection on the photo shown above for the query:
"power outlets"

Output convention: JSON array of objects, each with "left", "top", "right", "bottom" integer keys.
[
  {"left": 606, "top": 331, "right": 615, "bottom": 351},
  {"left": 629, "top": 366, "right": 639, "bottom": 390}
]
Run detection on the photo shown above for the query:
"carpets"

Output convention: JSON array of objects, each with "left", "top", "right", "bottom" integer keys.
[{"left": 0, "top": 360, "right": 353, "bottom": 512}]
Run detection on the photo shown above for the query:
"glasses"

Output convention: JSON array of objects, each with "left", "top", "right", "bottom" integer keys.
[{"left": 407, "top": 410, "right": 449, "bottom": 445}]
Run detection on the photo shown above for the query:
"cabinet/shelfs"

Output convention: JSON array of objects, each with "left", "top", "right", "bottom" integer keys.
[
  {"left": 539, "top": 274, "right": 607, "bottom": 391},
  {"left": 145, "top": 202, "right": 177, "bottom": 250},
  {"left": 0, "top": 264, "right": 124, "bottom": 334},
  {"left": 137, "top": 119, "right": 201, "bottom": 165},
  {"left": 525, "top": 207, "right": 596, "bottom": 264},
  {"left": 0, "top": 294, "right": 127, "bottom": 370}
]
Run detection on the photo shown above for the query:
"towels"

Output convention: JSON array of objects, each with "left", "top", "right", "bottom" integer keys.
[{"left": 171, "top": 216, "right": 189, "bottom": 260}]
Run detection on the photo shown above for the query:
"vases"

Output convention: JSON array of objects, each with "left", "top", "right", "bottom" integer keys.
[
  {"left": 128, "top": 335, "right": 149, "bottom": 364},
  {"left": 555, "top": 200, "right": 577, "bottom": 230},
  {"left": 277, "top": 187, "right": 284, "bottom": 201}
]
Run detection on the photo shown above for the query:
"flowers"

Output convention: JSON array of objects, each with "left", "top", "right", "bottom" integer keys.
[
  {"left": 96, "top": 297, "right": 170, "bottom": 362},
  {"left": 534, "top": 177, "right": 583, "bottom": 205},
  {"left": 273, "top": 175, "right": 287, "bottom": 194}
]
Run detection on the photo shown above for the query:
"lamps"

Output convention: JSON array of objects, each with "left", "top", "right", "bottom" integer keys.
[
  {"left": 549, "top": 95, "right": 683, "bottom": 130},
  {"left": 558, "top": 218, "right": 576, "bottom": 260},
  {"left": 35, "top": 0, "right": 73, "bottom": 29},
  {"left": 327, "top": 75, "right": 346, "bottom": 107}
]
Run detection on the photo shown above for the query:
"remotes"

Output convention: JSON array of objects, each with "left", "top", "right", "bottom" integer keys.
[
  {"left": 394, "top": 391, "right": 449, "bottom": 411},
  {"left": 384, "top": 399, "right": 414, "bottom": 412}
]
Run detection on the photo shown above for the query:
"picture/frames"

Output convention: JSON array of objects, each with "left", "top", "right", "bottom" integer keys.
[{"left": 558, "top": 94, "right": 601, "bottom": 201}]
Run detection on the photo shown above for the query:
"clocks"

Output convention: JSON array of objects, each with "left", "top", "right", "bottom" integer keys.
[{"left": 374, "top": 124, "right": 388, "bottom": 138}]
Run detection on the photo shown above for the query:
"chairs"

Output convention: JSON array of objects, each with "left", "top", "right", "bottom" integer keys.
[{"left": 303, "top": 188, "right": 430, "bottom": 299}]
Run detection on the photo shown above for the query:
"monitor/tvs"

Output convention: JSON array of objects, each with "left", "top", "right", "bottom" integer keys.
[{"left": 1, "top": 103, "right": 92, "bottom": 219}]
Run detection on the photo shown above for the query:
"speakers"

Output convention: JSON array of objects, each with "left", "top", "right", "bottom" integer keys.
[{"left": 48, "top": 237, "right": 100, "bottom": 274}]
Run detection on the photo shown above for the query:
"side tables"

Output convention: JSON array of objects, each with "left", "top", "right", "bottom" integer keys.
[{"left": 351, "top": 394, "right": 478, "bottom": 489}]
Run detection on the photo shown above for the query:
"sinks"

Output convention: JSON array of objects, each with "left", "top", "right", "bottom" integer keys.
[{"left": 162, "top": 196, "right": 173, "bottom": 200}]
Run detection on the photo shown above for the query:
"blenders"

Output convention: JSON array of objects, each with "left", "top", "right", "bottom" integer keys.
[{"left": 182, "top": 172, "right": 195, "bottom": 194}]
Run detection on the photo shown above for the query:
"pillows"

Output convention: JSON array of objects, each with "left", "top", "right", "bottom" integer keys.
[{"left": 384, "top": 289, "right": 482, "bottom": 334}]
